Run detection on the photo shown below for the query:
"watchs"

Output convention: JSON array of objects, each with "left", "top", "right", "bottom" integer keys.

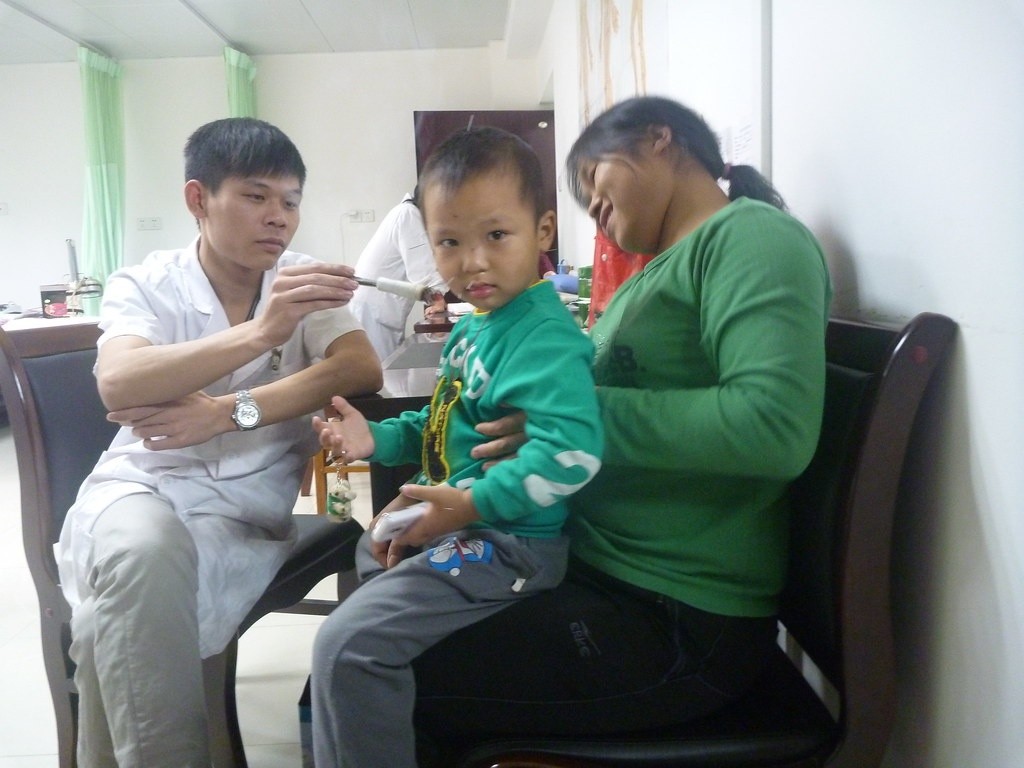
[{"left": 231, "top": 389, "right": 262, "bottom": 432}]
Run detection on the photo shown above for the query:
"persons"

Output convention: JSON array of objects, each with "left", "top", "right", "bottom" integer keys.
[
  {"left": 408, "top": 96, "right": 834, "bottom": 768},
  {"left": 309, "top": 118, "right": 605, "bottom": 768},
  {"left": 51, "top": 116, "right": 385, "bottom": 768},
  {"left": 347, "top": 184, "right": 451, "bottom": 363}
]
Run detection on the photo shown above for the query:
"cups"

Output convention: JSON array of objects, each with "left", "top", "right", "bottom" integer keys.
[
  {"left": 82, "top": 292, "right": 100, "bottom": 317},
  {"left": 578, "top": 265, "right": 593, "bottom": 328}
]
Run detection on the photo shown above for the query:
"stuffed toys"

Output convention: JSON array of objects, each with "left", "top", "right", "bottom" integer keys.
[{"left": 327, "top": 480, "right": 357, "bottom": 521}]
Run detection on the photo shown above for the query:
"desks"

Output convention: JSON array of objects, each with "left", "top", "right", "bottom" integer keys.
[
  {"left": 339, "top": 330, "right": 449, "bottom": 517},
  {"left": 414, "top": 311, "right": 450, "bottom": 333}
]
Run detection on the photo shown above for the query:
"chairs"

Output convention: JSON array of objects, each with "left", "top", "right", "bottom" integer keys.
[
  {"left": 0, "top": 323, "right": 364, "bottom": 768},
  {"left": 443, "top": 310, "right": 957, "bottom": 768},
  {"left": 300, "top": 404, "right": 370, "bottom": 517}
]
